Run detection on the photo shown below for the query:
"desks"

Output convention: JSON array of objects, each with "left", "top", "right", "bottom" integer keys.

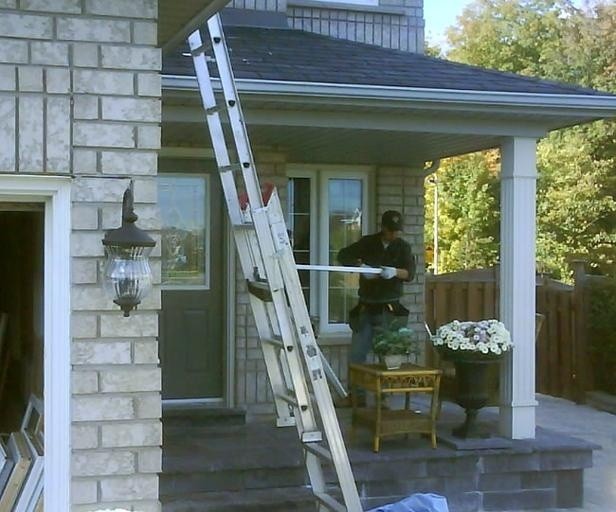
[{"left": 348, "top": 356, "right": 449, "bottom": 452}]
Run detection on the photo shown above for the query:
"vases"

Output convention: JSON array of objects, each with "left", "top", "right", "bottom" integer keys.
[{"left": 448, "top": 351, "right": 499, "bottom": 441}]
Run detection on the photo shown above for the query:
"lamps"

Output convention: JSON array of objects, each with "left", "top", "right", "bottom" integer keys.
[{"left": 98, "top": 183, "right": 158, "bottom": 319}]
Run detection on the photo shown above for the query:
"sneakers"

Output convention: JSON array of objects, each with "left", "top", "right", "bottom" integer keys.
[
  {"left": 335, "top": 394, "right": 366, "bottom": 407},
  {"left": 373, "top": 395, "right": 390, "bottom": 408}
]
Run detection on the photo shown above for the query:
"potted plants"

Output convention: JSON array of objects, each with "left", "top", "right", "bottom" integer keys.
[{"left": 371, "top": 318, "right": 421, "bottom": 371}]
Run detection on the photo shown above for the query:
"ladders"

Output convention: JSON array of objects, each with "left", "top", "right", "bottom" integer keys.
[{"left": 188, "top": 12, "right": 365, "bottom": 512}]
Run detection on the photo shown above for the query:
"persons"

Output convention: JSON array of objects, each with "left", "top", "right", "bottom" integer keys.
[{"left": 334, "top": 210, "right": 416, "bottom": 409}]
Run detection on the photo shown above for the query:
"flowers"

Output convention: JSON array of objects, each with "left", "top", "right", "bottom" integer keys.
[{"left": 424, "top": 316, "right": 516, "bottom": 358}]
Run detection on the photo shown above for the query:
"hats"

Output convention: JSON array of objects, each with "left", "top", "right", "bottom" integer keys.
[{"left": 382, "top": 210, "right": 405, "bottom": 231}]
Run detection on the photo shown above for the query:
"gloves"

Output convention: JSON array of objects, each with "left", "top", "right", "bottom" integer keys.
[
  {"left": 379, "top": 265, "right": 396, "bottom": 280},
  {"left": 360, "top": 264, "right": 379, "bottom": 280}
]
